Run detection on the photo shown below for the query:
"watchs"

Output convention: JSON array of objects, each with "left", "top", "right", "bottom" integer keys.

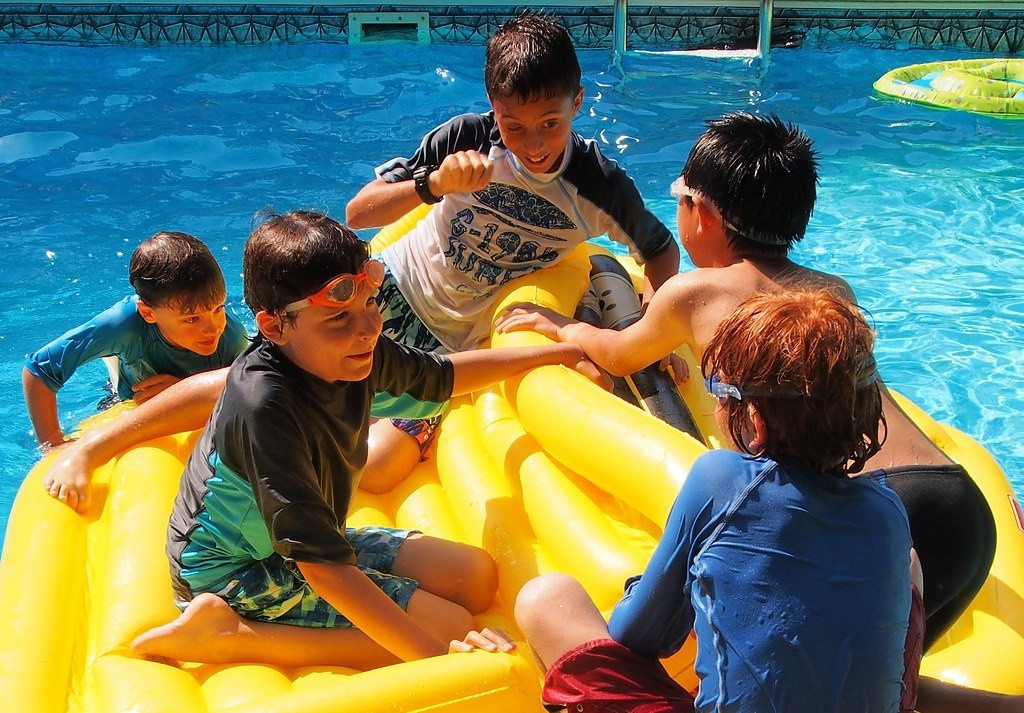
[{"left": 414, "top": 165, "right": 444, "bottom": 206}]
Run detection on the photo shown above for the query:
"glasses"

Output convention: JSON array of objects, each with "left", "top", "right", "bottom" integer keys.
[
  {"left": 670, "top": 174, "right": 791, "bottom": 244},
  {"left": 279, "top": 259, "right": 385, "bottom": 315},
  {"left": 706, "top": 371, "right": 882, "bottom": 401}
]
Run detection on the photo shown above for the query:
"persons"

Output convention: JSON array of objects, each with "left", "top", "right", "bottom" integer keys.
[
  {"left": 45, "top": 15, "right": 690, "bottom": 514},
  {"left": 514, "top": 285, "right": 928, "bottom": 713},
  {"left": 493, "top": 112, "right": 1024, "bottom": 713},
  {"left": 24, "top": 230, "right": 250, "bottom": 450},
  {"left": 132, "top": 209, "right": 614, "bottom": 674}
]
